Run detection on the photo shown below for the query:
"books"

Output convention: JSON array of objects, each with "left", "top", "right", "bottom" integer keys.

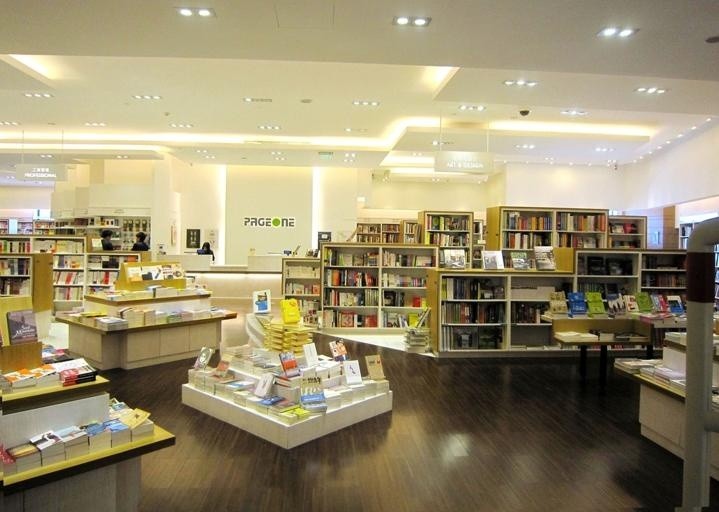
[
  {"left": 0, "top": 217, "right": 223, "bottom": 477},
  {"left": 285, "top": 213, "right": 718, "bottom": 405},
  {"left": 223, "top": 343, "right": 328, "bottom": 427}
]
